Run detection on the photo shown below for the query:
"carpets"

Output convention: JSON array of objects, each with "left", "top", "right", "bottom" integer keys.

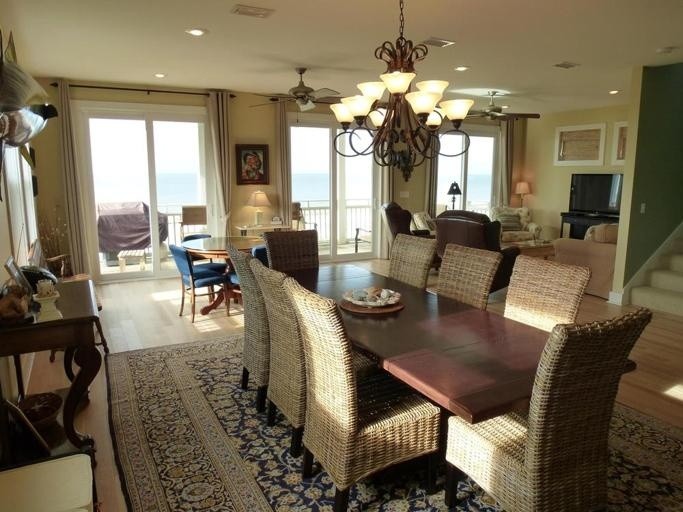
[{"left": 105, "top": 336, "right": 682, "bottom": 512}]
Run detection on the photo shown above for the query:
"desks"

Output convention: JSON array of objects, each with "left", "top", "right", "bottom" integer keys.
[
  {"left": 0, "top": 280, "right": 103, "bottom": 448},
  {"left": 97, "top": 201, "right": 168, "bottom": 266}
]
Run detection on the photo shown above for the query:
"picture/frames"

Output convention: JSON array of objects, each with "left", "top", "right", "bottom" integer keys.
[
  {"left": 5, "top": 256, "right": 34, "bottom": 298},
  {"left": 553, "top": 121, "right": 629, "bottom": 166},
  {"left": 235, "top": 144, "right": 268, "bottom": 185}
]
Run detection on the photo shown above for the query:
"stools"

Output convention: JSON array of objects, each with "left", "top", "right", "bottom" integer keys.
[
  {"left": 17, "top": 392, "right": 67, "bottom": 448},
  {"left": 0, "top": 399, "right": 97, "bottom": 512},
  {"left": 19, "top": 265, "right": 109, "bottom": 363},
  {"left": 117, "top": 249, "right": 146, "bottom": 272}
]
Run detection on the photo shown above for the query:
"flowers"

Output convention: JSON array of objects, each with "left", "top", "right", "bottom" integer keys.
[{"left": 39, "top": 204, "right": 68, "bottom": 259}]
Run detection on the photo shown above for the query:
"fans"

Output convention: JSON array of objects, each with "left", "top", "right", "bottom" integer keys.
[
  {"left": 467, "top": 91, "right": 540, "bottom": 119},
  {"left": 248, "top": 67, "right": 340, "bottom": 112}
]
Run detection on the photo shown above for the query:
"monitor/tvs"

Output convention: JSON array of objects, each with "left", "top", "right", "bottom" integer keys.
[{"left": 568, "top": 173, "right": 624, "bottom": 217}]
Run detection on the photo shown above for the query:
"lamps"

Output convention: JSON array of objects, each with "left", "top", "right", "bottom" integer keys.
[
  {"left": 447, "top": 181, "right": 462, "bottom": 210},
  {"left": 329, "top": 0, "right": 474, "bottom": 182},
  {"left": 515, "top": 182, "right": 530, "bottom": 208},
  {"left": 244, "top": 191, "right": 271, "bottom": 225}
]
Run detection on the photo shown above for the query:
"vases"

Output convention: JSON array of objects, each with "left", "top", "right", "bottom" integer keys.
[{"left": 47, "top": 253, "right": 72, "bottom": 277}]
